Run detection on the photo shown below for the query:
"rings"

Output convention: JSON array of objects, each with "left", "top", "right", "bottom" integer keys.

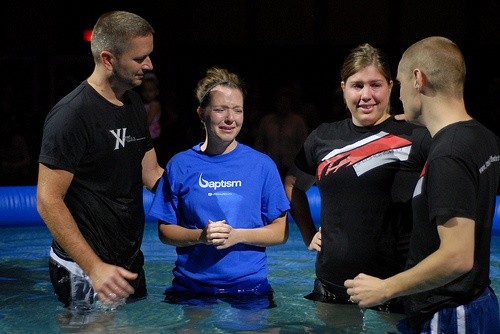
[{"left": 108, "top": 292, "right": 114, "bottom": 298}]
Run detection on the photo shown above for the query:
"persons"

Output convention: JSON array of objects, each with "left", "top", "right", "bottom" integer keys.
[
  {"left": 148, "top": 68, "right": 290, "bottom": 322},
  {"left": 35, "top": 12, "right": 166, "bottom": 315},
  {"left": 344, "top": 36, "right": 500, "bottom": 334},
  {"left": 284, "top": 42, "right": 432, "bottom": 334}
]
